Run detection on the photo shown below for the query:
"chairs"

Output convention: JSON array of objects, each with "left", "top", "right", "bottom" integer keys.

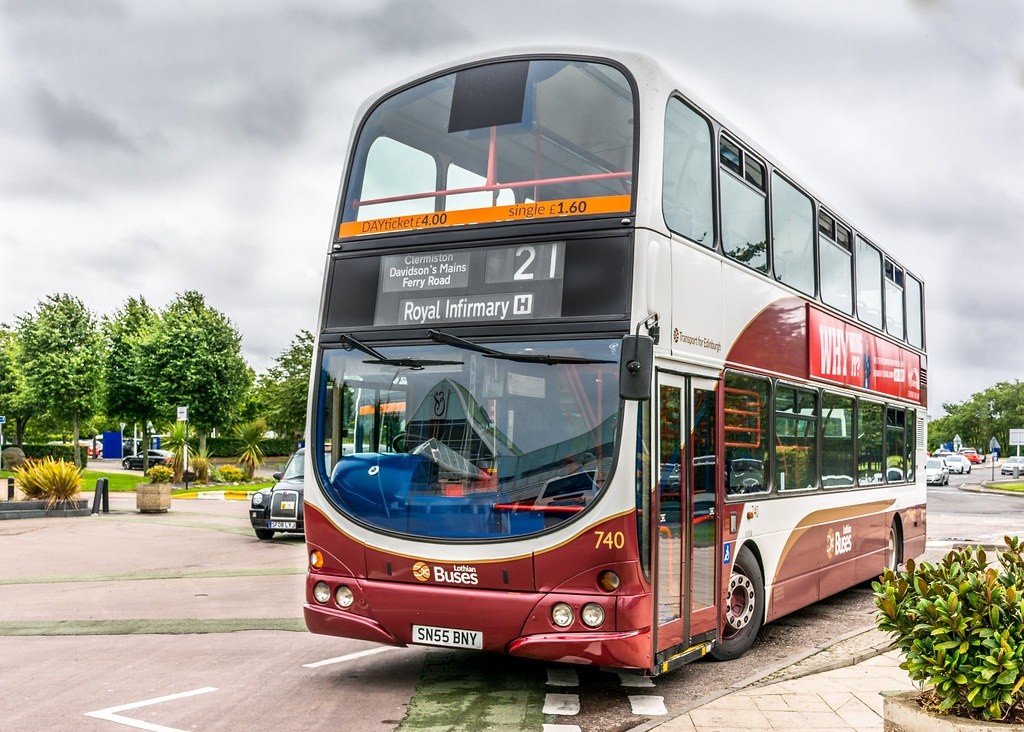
[
  {"left": 829, "top": 294, "right": 921, "bottom": 347},
  {"left": 660, "top": 456, "right": 764, "bottom": 523}
]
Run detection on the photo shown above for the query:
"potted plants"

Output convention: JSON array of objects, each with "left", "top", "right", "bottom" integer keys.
[
  {"left": 870, "top": 536, "right": 1024, "bottom": 732},
  {"left": 136, "top": 465, "right": 175, "bottom": 513}
]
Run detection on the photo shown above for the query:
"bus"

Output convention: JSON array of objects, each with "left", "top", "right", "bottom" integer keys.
[{"left": 299, "top": 42, "right": 933, "bottom": 676}]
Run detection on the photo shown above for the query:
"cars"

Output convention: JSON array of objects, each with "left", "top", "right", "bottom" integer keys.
[
  {"left": 930, "top": 447, "right": 985, "bottom": 465},
  {"left": 924, "top": 458, "right": 951, "bottom": 486},
  {"left": 247, "top": 443, "right": 396, "bottom": 541},
  {"left": 943, "top": 455, "right": 973, "bottom": 475},
  {"left": 121, "top": 449, "right": 175, "bottom": 470},
  {"left": 47, "top": 439, "right": 103, "bottom": 457},
  {"left": 1001, "top": 456, "right": 1024, "bottom": 475}
]
